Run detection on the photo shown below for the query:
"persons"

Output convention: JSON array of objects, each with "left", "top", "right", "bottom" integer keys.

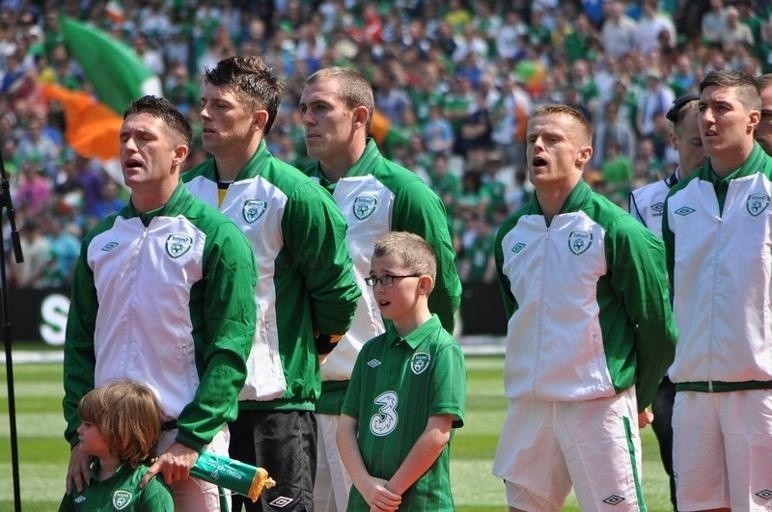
[
  {"left": 626, "top": 92, "right": 706, "bottom": 511},
  {"left": 61, "top": 94, "right": 258, "bottom": 512},
  {"left": 297, "top": 66, "right": 465, "bottom": 512},
  {"left": 58, "top": 380, "right": 175, "bottom": 512},
  {"left": 1, "top": 1, "right": 772, "bottom": 286},
  {"left": 336, "top": 231, "right": 468, "bottom": 512},
  {"left": 490, "top": 103, "right": 679, "bottom": 511},
  {"left": 176, "top": 55, "right": 361, "bottom": 512},
  {"left": 752, "top": 73, "right": 771, "bottom": 156},
  {"left": 659, "top": 70, "right": 772, "bottom": 512}
]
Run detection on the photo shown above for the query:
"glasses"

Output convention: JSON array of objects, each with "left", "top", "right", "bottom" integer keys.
[{"left": 364, "top": 275, "right": 420, "bottom": 286}]
